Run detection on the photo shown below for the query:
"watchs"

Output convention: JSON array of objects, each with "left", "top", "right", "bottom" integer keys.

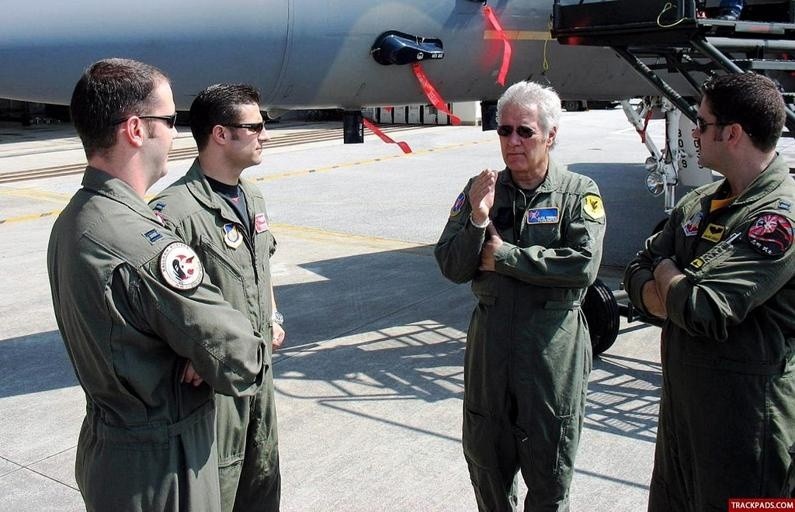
[{"left": 270, "top": 311, "right": 284, "bottom": 326}]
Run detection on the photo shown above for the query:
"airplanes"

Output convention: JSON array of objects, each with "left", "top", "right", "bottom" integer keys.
[{"left": 0, "top": 1, "right": 794, "bottom": 368}]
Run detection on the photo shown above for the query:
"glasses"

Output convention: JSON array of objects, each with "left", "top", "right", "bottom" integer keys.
[
  {"left": 222, "top": 121, "right": 267, "bottom": 135},
  {"left": 497, "top": 123, "right": 535, "bottom": 140},
  {"left": 112, "top": 111, "right": 179, "bottom": 130},
  {"left": 696, "top": 116, "right": 719, "bottom": 135}
]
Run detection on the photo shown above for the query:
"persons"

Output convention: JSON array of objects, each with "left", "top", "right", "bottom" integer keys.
[
  {"left": 148, "top": 83, "right": 287, "bottom": 512},
  {"left": 622, "top": 73, "right": 795, "bottom": 511},
  {"left": 433, "top": 79, "right": 605, "bottom": 511},
  {"left": 44, "top": 56, "right": 270, "bottom": 512}
]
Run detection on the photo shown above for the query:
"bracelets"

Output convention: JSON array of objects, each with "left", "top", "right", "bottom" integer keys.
[{"left": 469, "top": 211, "right": 490, "bottom": 229}]
